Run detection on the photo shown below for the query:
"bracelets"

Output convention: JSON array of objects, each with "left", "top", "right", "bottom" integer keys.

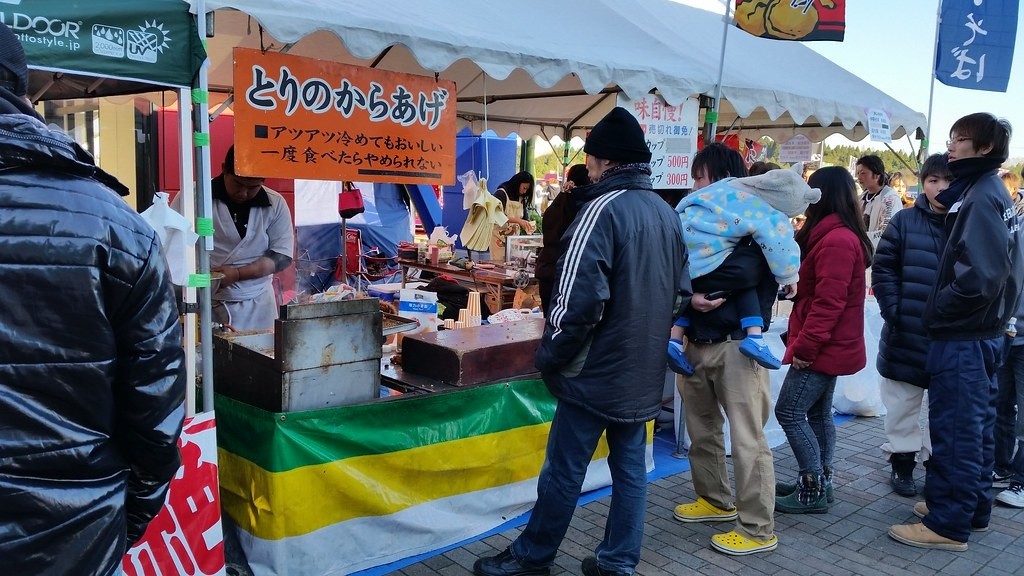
[{"left": 235, "top": 268, "right": 241, "bottom": 282}]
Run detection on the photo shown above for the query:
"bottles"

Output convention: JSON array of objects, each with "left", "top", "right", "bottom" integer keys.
[
  {"left": 418, "top": 224, "right": 453, "bottom": 266},
  {"left": 1006, "top": 316, "right": 1017, "bottom": 336}
]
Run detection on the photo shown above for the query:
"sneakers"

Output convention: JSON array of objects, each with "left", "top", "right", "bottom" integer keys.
[
  {"left": 888, "top": 502, "right": 988, "bottom": 551},
  {"left": 891, "top": 453, "right": 918, "bottom": 496},
  {"left": 775, "top": 471, "right": 834, "bottom": 514},
  {"left": 991, "top": 470, "right": 1024, "bottom": 509}
]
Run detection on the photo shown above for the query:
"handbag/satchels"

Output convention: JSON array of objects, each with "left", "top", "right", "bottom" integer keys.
[{"left": 338, "top": 181, "right": 365, "bottom": 218}]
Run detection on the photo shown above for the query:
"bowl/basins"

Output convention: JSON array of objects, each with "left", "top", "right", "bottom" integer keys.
[{"left": 210, "top": 272, "right": 225, "bottom": 298}]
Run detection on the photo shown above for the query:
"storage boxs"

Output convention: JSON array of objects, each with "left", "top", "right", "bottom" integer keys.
[
  {"left": 425, "top": 252, "right": 453, "bottom": 258},
  {"left": 432, "top": 247, "right": 439, "bottom": 265},
  {"left": 398, "top": 246, "right": 418, "bottom": 260},
  {"left": 367, "top": 281, "right": 430, "bottom": 304},
  {"left": 397, "top": 289, "right": 438, "bottom": 352},
  {"left": 506, "top": 235, "right": 544, "bottom": 262}
]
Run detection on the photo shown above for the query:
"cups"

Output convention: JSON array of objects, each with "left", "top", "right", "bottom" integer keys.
[{"left": 444, "top": 291, "right": 481, "bottom": 330}]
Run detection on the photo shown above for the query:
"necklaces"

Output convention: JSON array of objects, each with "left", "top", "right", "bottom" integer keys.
[{"left": 866, "top": 184, "right": 884, "bottom": 202}]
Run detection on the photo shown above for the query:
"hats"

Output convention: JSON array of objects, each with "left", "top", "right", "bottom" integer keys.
[
  {"left": 0, "top": 23, "right": 28, "bottom": 97},
  {"left": 583, "top": 107, "right": 652, "bottom": 163}
]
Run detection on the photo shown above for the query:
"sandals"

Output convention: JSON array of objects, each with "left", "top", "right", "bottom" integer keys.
[
  {"left": 740, "top": 338, "right": 782, "bottom": 370},
  {"left": 668, "top": 343, "right": 693, "bottom": 377}
]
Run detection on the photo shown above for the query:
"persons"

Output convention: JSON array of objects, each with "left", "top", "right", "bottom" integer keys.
[
  {"left": 172, "top": 143, "right": 295, "bottom": 351},
  {"left": 474, "top": 104, "right": 1023, "bottom": 576},
  {"left": 0, "top": 23, "right": 189, "bottom": 576}
]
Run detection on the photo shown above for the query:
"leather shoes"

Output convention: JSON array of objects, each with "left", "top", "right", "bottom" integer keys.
[
  {"left": 582, "top": 557, "right": 612, "bottom": 576},
  {"left": 473, "top": 546, "right": 551, "bottom": 576}
]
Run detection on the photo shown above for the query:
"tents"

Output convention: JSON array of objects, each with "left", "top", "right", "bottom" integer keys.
[
  {"left": 190, "top": 1, "right": 928, "bottom": 457},
  {"left": 0, "top": 0, "right": 208, "bottom": 423}
]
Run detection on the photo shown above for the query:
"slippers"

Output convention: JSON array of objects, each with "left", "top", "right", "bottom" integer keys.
[
  {"left": 711, "top": 528, "right": 779, "bottom": 556},
  {"left": 673, "top": 496, "right": 739, "bottom": 522}
]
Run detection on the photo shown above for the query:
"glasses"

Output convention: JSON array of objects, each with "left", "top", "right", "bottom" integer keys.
[{"left": 946, "top": 136, "right": 974, "bottom": 147}]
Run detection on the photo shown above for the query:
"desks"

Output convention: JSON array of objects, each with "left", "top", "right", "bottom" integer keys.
[{"left": 394, "top": 255, "right": 539, "bottom": 314}]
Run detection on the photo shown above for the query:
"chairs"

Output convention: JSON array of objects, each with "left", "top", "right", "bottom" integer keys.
[
  {"left": 273, "top": 228, "right": 306, "bottom": 306},
  {"left": 336, "top": 228, "right": 402, "bottom": 294},
  {"left": 295, "top": 248, "right": 338, "bottom": 294}
]
